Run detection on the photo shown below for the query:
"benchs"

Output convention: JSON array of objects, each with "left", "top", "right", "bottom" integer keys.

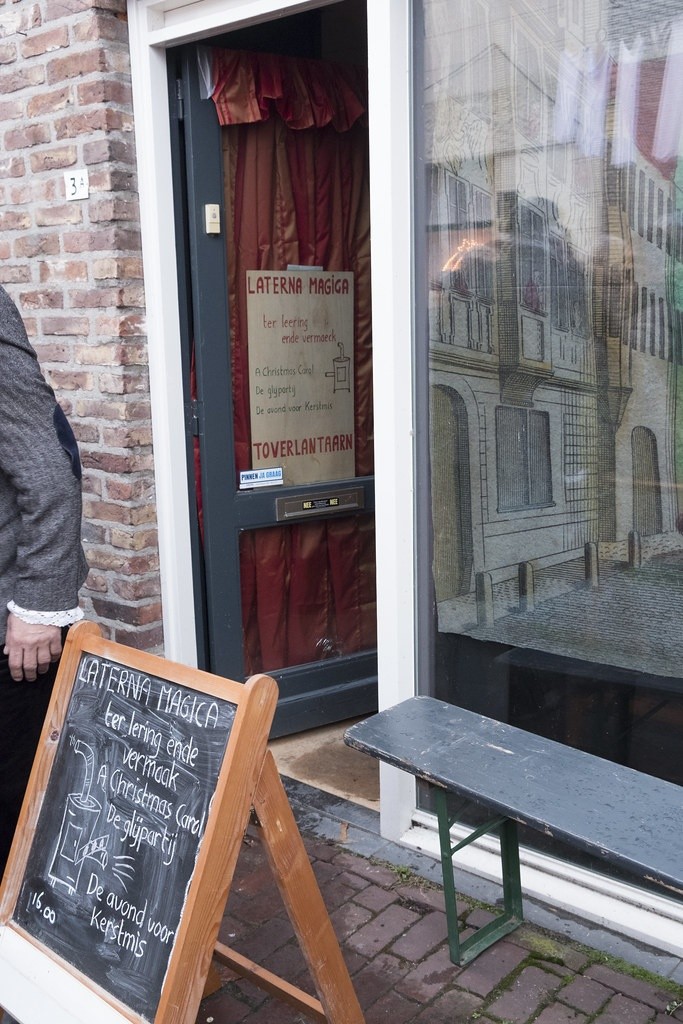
[{"left": 342, "top": 695, "right": 682, "bottom": 969}]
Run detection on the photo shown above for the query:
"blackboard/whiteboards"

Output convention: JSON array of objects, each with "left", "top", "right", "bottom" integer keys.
[{"left": 0, "top": 619, "right": 368, "bottom": 1024}]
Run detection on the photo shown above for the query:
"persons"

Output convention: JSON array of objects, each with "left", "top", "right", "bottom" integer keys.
[{"left": 0, "top": 282, "right": 89, "bottom": 880}]
